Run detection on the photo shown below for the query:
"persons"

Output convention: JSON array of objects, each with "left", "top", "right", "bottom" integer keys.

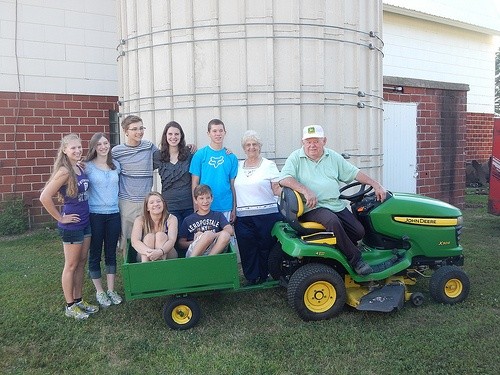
[
  {"left": 130, "top": 191, "right": 177, "bottom": 262},
  {"left": 39, "top": 135, "right": 100, "bottom": 320},
  {"left": 76, "top": 132, "right": 123, "bottom": 305},
  {"left": 176, "top": 185, "right": 232, "bottom": 259},
  {"left": 226, "top": 130, "right": 280, "bottom": 286},
  {"left": 76, "top": 116, "right": 197, "bottom": 262},
  {"left": 153, "top": 122, "right": 195, "bottom": 248},
  {"left": 280, "top": 125, "right": 387, "bottom": 275},
  {"left": 189, "top": 119, "right": 238, "bottom": 227}
]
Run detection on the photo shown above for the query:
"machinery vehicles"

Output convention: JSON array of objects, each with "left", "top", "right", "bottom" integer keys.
[{"left": 120, "top": 182, "right": 471, "bottom": 333}]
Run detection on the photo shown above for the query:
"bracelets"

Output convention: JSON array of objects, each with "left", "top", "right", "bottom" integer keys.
[{"left": 160, "top": 248, "right": 165, "bottom": 255}]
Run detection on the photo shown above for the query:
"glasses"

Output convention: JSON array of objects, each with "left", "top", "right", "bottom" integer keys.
[{"left": 126, "top": 126, "right": 146, "bottom": 132}]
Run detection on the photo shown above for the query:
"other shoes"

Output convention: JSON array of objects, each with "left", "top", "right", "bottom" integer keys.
[
  {"left": 352, "top": 256, "right": 372, "bottom": 275},
  {"left": 246, "top": 279, "right": 256, "bottom": 286},
  {"left": 271, "top": 274, "right": 280, "bottom": 282},
  {"left": 257, "top": 276, "right": 266, "bottom": 284}
]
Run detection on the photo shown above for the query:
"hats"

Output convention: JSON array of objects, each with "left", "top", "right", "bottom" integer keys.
[{"left": 303, "top": 125, "right": 325, "bottom": 139}]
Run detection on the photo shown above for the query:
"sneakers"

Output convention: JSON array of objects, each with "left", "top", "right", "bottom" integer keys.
[
  {"left": 107, "top": 290, "right": 123, "bottom": 305},
  {"left": 97, "top": 292, "right": 112, "bottom": 307},
  {"left": 65, "top": 304, "right": 91, "bottom": 320},
  {"left": 75, "top": 299, "right": 99, "bottom": 313}
]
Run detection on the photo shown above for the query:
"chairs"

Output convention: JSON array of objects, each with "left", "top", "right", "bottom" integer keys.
[{"left": 277, "top": 186, "right": 337, "bottom": 246}]
